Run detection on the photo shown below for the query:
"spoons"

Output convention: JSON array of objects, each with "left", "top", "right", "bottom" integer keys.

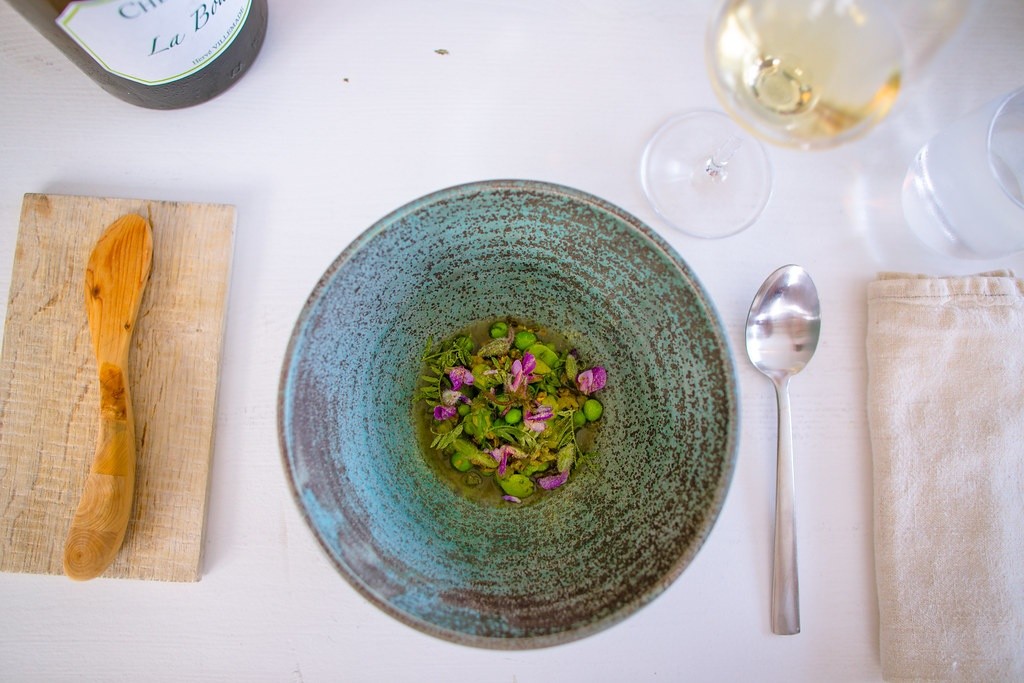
[{"left": 746, "top": 263, "right": 822, "bottom": 636}]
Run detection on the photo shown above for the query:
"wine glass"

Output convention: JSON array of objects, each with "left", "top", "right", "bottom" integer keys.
[{"left": 642, "top": 0, "right": 970, "bottom": 239}]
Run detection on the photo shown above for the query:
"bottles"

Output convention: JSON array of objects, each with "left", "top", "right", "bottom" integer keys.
[{"left": 5, "top": 0, "right": 269, "bottom": 111}]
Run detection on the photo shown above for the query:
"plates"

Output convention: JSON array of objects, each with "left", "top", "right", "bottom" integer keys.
[{"left": 274, "top": 179, "right": 741, "bottom": 648}]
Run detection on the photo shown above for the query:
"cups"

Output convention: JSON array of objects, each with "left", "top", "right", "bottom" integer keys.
[{"left": 902, "top": 88, "right": 1024, "bottom": 265}]
